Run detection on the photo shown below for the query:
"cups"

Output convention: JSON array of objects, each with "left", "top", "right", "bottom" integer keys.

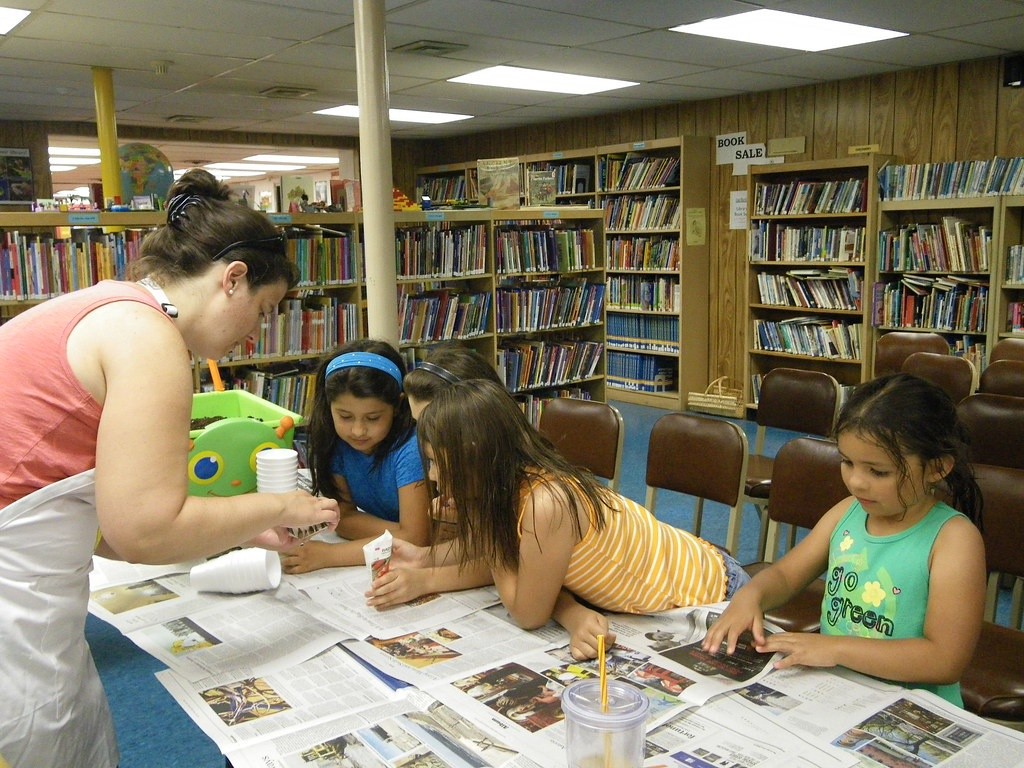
[
  {"left": 561, "top": 678, "right": 652, "bottom": 768},
  {"left": 255, "top": 449, "right": 298, "bottom": 492},
  {"left": 190, "top": 547, "right": 282, "bottom": 594}
]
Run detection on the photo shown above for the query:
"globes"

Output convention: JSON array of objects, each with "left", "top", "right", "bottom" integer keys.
[{"left": 119, "top": 142, "right": 174, "bottom": 206}]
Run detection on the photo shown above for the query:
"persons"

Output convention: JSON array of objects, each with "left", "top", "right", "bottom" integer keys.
[
  {"left": 417, "top": 379, "right": 752, "bottom": 660},
  {"left": 364, "top": 341, "right": 505, "bottom": 612},
  {"left": 701, "top": 373, "right": 987, "bottom": 710},
  {"left": 645, "top": 609, "right": 700, "bottom": 645},
  {"left": 467, "top": 665, "right": 565, "bottom": 727},
  {"left": 275, "top": 340, "right": 431, "bottom": 575},
  {"left": 0, "top": 168, "right": 300, "bottom": 768}
]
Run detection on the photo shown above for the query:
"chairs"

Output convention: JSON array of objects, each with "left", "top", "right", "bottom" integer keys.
[{"left": 539, "top": 331, "right": 1024, "bottom": 732}]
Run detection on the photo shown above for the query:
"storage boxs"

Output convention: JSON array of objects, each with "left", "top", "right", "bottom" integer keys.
[{"left": 186, "top": 388, "right": 304, "bottom": 497}]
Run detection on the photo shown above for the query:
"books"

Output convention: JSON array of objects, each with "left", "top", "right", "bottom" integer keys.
[
  {"left": 751, "top": 156, "right": 1024, "bottom": 405},
  {"left": 395, "top": 152, "right": 680, "bottom": 431},
  {"left": 0, "top": 228, "right": 368, "bottom": 418}
]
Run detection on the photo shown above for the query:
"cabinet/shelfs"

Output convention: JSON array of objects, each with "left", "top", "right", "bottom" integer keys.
[
  {"left": 0, "top": 133, "right": 711, "bottom": 468},
  {"left": 742, "top": 156, "right": 1024, "bottom": 420}
]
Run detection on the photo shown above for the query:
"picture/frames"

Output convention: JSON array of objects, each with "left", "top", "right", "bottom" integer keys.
[{"left": 1, "top": 147, "right": 35, "bottom": 206}]
